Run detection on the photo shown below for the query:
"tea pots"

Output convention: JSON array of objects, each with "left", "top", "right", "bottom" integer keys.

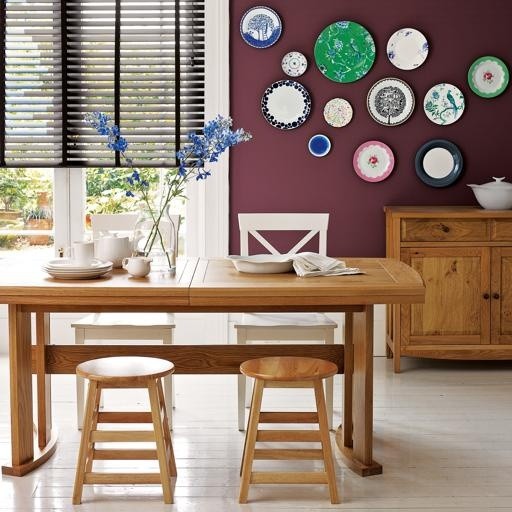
[{"left": 96, "top": 231, "right": 145, "bottom": 268}]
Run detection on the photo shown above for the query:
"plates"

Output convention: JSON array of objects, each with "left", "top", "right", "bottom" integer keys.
[
  {"left": 385, "top": 28, "right": 429, "bottom": 73},
  {"left": 281, "top": 51, "right": 309, "bottom": 78},
  {"left": 241, "top": 5, "right": 281, "bottom": 50},
  {"left": 307, "top": 79, "right": 467, "bottom": 190},
  {"left": 44, "top": 260, "right": 113, "bottom": 279},
  {"left": 466, "top": 55, "right": 510, "bottom": 98},
  {"left": 260, "top": 79, "right": 312, "bottom": 131},
  {"left": 313, "top": 20, "right": 376, "bottom": 83}
]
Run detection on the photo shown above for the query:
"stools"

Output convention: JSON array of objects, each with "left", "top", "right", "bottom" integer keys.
[
  {"left": 71, "top": 354, "right": 179, "bottom": 508},
  {"left": 237, "top": 356, "right": 344, "bottom": 506}
]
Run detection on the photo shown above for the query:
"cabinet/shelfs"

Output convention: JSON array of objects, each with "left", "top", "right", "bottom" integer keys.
[{"left": 382, "top": 202, "right": 511, "bottom": 375}]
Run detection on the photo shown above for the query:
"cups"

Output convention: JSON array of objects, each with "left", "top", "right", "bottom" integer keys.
[
  {"left": 66, "top": 241, "right": 95, "bottom": 265},
  {"left": 120, "top": 256, "right": 153, "bottom": 277}
]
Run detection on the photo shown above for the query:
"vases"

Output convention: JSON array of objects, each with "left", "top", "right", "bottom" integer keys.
[{"left": 130, "top": 207, "right": 182, "bottom": 273}]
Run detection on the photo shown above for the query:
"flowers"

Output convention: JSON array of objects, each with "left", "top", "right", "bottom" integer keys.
[{"left": 79, "top": 107, "right": 254, "bottom": 267}]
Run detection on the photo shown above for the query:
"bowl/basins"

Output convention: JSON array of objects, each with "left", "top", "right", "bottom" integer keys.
[
  {"left": 224, "top": 252, "right": 294, "bottom": 276},
  {"left": 465, "top": 175, "right": 511, "bottom": 209}
]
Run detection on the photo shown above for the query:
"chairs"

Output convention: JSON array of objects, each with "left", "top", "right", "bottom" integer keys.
[
  {"left": 71, "top": 209, "right": 186, "bottom": 435},
  {"left": 234, "top": 209, "right": 341, "bottom": 441}
]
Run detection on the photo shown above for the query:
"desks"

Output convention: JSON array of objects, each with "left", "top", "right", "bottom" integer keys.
[{"left": 0, "top": 254, "right": 428, "bottom": 481}]
